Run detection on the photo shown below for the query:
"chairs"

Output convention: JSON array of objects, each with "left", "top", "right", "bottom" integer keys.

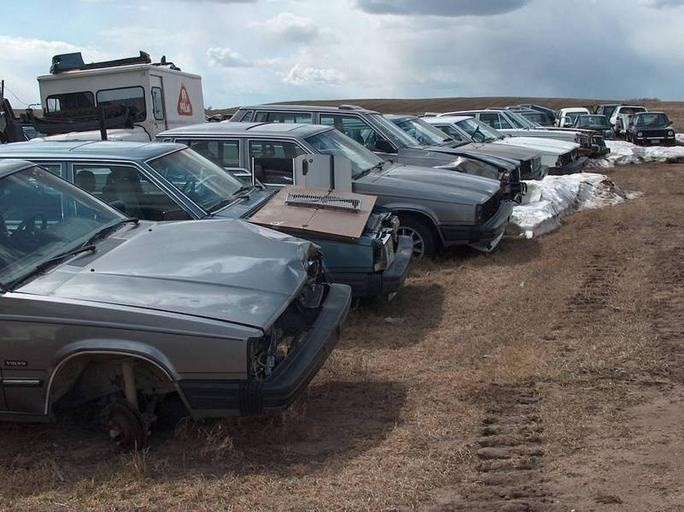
[{"left": 75, "top": 167, "right": 144, "bottom": 218}]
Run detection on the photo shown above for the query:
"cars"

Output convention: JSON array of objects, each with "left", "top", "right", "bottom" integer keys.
[
  {"left": 215, "top": 104, "right": 524, "bottom": 203},
  {"left": 0, "top": 159, "right": 352, "bottom": 454},
  {"left": 150, "top": 121, "right": 523, "bottom": 266},
  {"left": 385, "top": 103, "right": 677, "bottom": 180},
  {"left": 0, "top": 139, "right": 412, "bottom": 298}
]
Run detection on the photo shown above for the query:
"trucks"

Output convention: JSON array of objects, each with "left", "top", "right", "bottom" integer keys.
[{"left": 1, "top": 51, "right": 205, "bottom": 142}]
